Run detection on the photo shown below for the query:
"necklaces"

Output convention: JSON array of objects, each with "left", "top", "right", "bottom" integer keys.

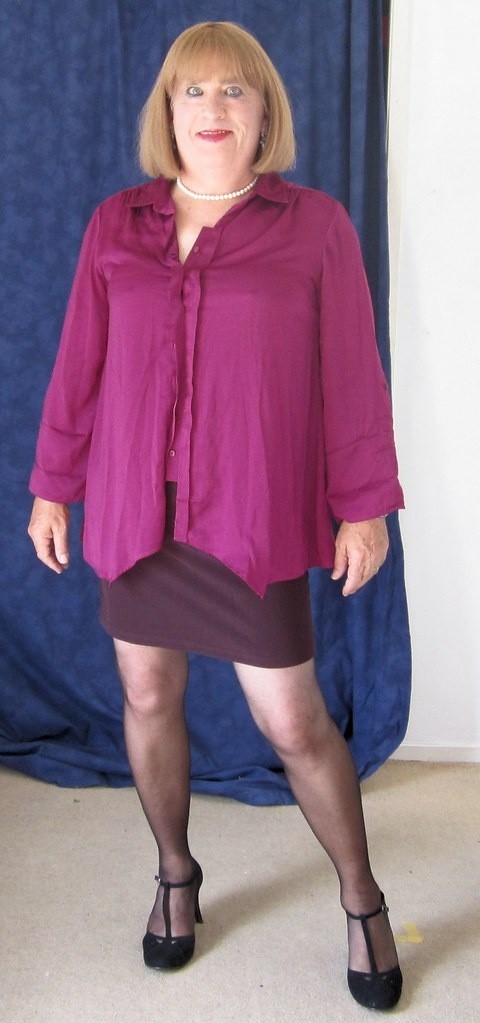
[{"left": 177, "top": 174, "right": 257, "bottom": 200}]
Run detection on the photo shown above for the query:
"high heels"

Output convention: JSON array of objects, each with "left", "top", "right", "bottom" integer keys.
[
  {"left": 346, "top": 891, "right": 403, "bottom": 1012},
  {"left": 143, "top": 855, "right": 204, "bottom": 972}
]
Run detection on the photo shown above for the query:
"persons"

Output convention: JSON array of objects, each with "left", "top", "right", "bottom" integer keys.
[{"left": 28, "top": 21, "right": 403, "bottom": 1012}]
